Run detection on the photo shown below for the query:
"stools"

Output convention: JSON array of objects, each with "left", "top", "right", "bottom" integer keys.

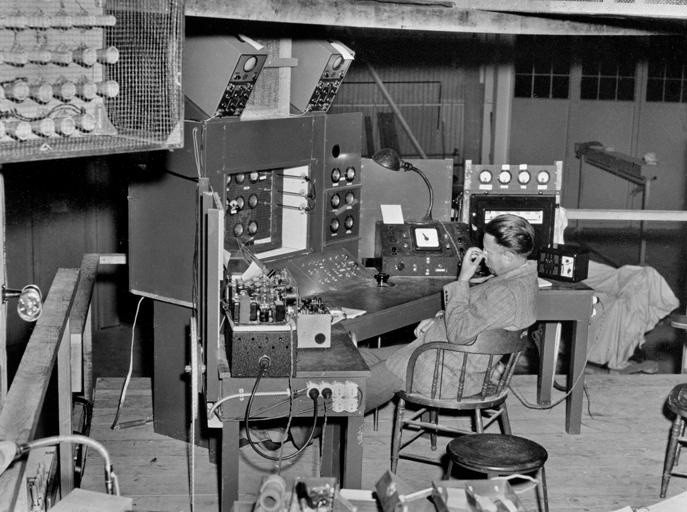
[
  {"left": 443, "top": 433, "right": 549, "bottom": 512},
  {"left": 660, "top": 383, "right": 687, "bottom": 498}
]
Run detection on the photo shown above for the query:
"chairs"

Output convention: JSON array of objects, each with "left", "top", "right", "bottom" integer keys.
[{"left": 391, "top": 328, "right": 528, "bottom": 475}]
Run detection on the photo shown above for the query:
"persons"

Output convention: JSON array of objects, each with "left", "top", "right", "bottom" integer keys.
[{"left": 291, "top": 214, "right": 539, "bottom": 449}]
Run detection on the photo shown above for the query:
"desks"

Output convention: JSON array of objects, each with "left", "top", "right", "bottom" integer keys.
[{"left": 220, "top": 275, "right": 595, "bottom": 511}]
